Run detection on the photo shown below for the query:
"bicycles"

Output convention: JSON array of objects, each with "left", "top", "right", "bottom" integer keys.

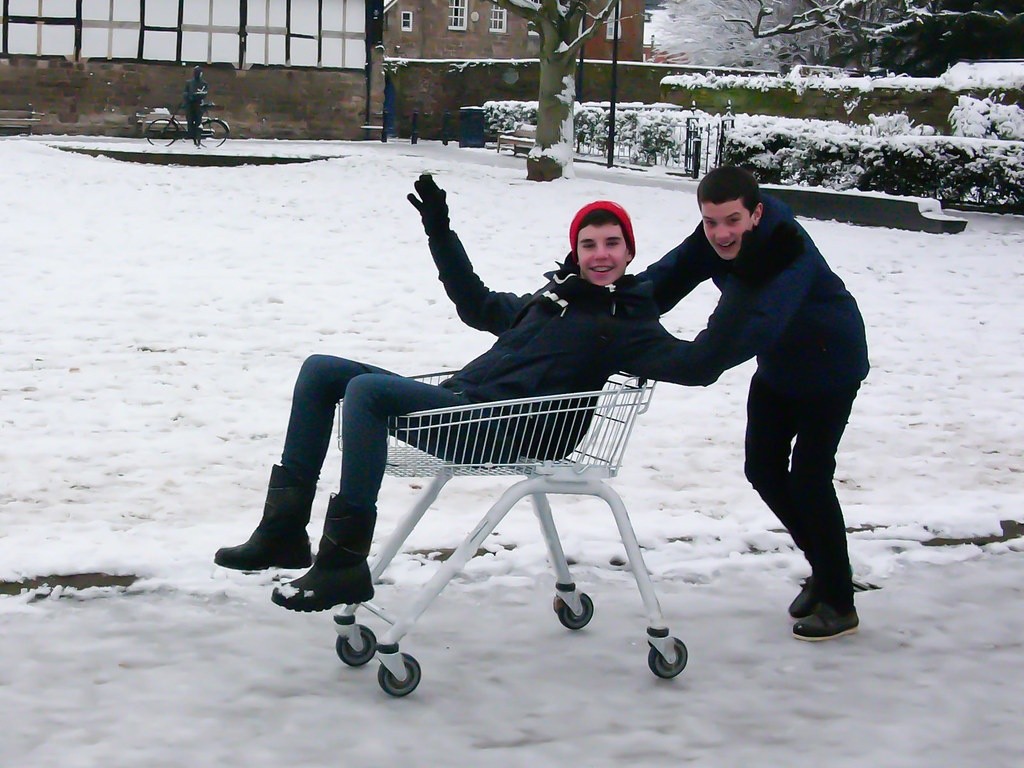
[{"left": 144, "top": 98, "right": 231, "bottom": 149}]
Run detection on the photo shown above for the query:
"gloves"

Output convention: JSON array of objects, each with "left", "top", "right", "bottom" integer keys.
[
  {"left": 737, "top": 221, "right": 805, "bottom": 279},
  {"left": 407, "top": 173, "right": 451, "bottom": 237}
]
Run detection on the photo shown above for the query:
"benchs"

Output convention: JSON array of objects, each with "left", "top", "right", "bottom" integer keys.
[
  {"left": 495, "top": 121, "right": 536, "bottom": 156},
  {"left": 135, "top": 112, "right": 216, "bottom": 137},
  {"left": 0, "top": 103, "right": 45, "bottom": 135}
]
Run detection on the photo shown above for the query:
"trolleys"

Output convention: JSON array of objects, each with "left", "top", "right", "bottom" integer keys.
[{"left": 335, "top": 361, "right": 690, "bottom": 697}]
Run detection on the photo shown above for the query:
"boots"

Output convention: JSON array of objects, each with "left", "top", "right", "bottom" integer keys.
[
  {"left": 271, "top": 492, "right": 378, "bottom": 612},
  {"left": 213, "top": 463, "right": 318, "bottom": 571}
]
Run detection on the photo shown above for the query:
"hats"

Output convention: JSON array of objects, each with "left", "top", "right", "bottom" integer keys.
[{"left": 570, "top": 201, "right": 635, "bottom": 266}]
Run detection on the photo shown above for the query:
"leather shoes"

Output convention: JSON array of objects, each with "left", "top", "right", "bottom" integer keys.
[
  {"left": 792, "top": 602, "right": 859, "bottom": 641},
  {"left": 788, "top": 577, "right": 822, "bottom": 621}
]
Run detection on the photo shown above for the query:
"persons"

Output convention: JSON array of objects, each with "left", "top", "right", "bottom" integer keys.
[
  {"left": 185, "top": 67, "right": 208, "bottom": 148},
  {"left": 618, "top": 166, "right": 869, "bottom": 641},
  {"left": 213, "top": 172, "right": 637, "bottom": 612}
]
